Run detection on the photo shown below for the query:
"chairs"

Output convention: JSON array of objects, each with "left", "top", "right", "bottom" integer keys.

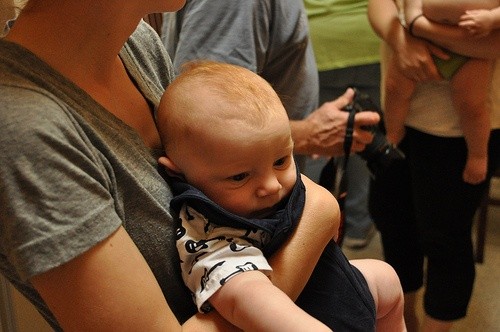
[{"left": 474, "top": 126, "right": 500, "bottom": 264}]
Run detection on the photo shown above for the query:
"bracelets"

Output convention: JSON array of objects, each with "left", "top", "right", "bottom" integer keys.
[{"left": 408, "top": 13, "right": 428, "bottom": 37}]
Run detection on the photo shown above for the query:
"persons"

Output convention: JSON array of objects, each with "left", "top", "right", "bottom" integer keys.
[
  {"left": 0, "top": 0, "right": 340, "bottom": 332},
  {"left": 158, "top": 58, "right": 407, "bottom": 332},
  {"left": 366, "top": 0, "right": 500, "bottom": 332},
  {"left": 383, "top": 0, "right": 500, "bottom": 184},
  {"left": 150, "top": 0, "right": 394, "bottom": 252}
]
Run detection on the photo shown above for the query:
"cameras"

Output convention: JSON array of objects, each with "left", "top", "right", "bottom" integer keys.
[{"left": 340, "top": 84, "right": 406, "bottom": 184}]
